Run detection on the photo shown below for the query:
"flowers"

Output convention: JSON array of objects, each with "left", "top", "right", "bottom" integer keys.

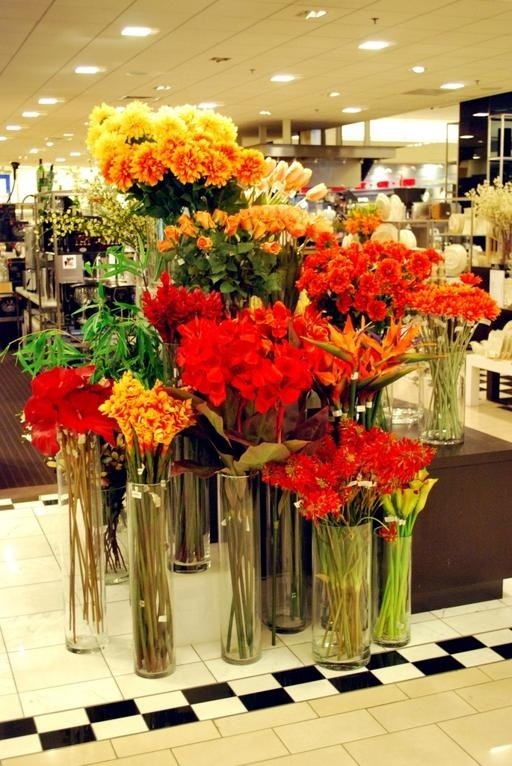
[
  {"left": 15, "top": 363, "right": 121, "bottom": 460},
  {"left": 83, "top": 99, "right": 501, "bottom": 328},
  {"left": 464, "top": 177, "right": 512, "bottom": 263},
  {"left": 148, "top": 263, "right": 434, "bottom": 522},
  {"left": 95, "top": 370, "right": 199, "bottom": 479}
]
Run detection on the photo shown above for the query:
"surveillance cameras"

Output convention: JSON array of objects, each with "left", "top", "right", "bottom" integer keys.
[{"left": 12, "top": 161, "right": 19, "bottom": 169}]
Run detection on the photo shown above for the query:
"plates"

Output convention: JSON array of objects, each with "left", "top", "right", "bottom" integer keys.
[{"left": 372, "top": 193, "right": 468, "bottom": 278}]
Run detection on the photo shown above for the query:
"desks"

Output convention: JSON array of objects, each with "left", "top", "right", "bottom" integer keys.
[{"left": 382, "top": 397, "right": 512, "bottom": 618}]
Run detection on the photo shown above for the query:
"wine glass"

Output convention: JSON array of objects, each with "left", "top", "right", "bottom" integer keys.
[{"left": 73, "top": 287, "right": 107, "bottom": 325}]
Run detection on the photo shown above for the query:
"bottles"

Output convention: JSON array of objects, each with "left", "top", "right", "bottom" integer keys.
[
  {"left": 73, "top": 196, "right": 80, "bottom": 209},
  {"left": 36, "top": 157, "right": 55, "bottom": 193}
]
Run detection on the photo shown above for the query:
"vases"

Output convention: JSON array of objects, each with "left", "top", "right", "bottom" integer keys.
[
  {"left": 310, "top": 515, "right": 378, "bottom": 672},
  {"left": 56, "top": 422, "right": 115, "bottom": 655},
  {"left": 256, "top": 467, "right": 312, "bottom": 636},
  {"left": 166, "top": 459, "right": 214, "bottom": 578},
  {"left": 372, "top": 523, "right": 417, "bottom": 650},
  {"left": 126, "top": 480, "right": 177, "bottom": 679},
  {"left": 211, "top": 468, "right": 266, "bottom": 667},
  {"left": 91, "top": 480, "right": 136, "bottom": 587},
  {"left": 408, "top": 335, "right": 477, "bottom": 448}
]
